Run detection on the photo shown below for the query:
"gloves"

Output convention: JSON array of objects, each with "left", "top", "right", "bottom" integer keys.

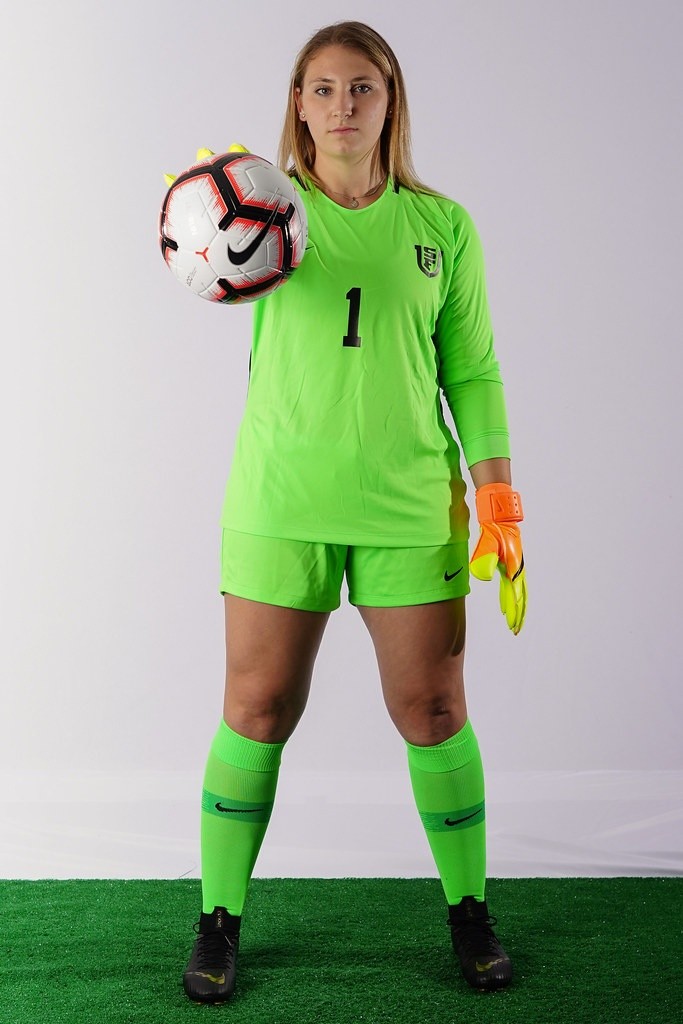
[
  {"left": 163, "top": 145, "right": 249, "bottom": 191},
  {"left": 472, "top": 483, "right": 527, "bottom": 636}
]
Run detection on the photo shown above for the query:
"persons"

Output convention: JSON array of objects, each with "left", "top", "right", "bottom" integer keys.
[{"left": 165, "top": 22, "right": 527, "bottom": 1007}]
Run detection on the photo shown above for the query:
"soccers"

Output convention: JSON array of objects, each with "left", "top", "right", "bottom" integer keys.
[{"left": 158, "top": 154, "right": 311, "bottom": 303}]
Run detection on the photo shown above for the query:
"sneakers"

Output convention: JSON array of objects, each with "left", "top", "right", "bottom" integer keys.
[
  {"left": 449, "top": 896, "right": 511, "bottom": 992},
  {"left": 183, "top": 906, "right": 241, "bottom": 1003}
]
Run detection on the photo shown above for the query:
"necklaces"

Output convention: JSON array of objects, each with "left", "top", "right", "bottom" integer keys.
[{"left": 321, "top": 173, "right": 387, "bottom": 208}]
